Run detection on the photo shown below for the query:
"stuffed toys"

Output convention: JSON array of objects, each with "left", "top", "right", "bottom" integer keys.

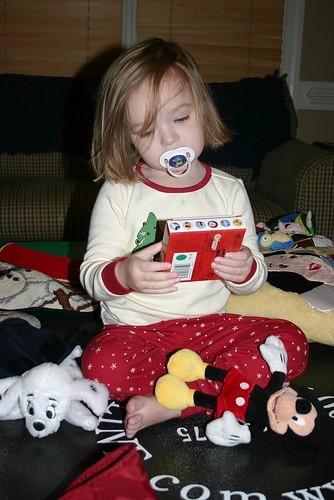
[
  {"left": 0, "top": 345, "right": 110, "bottom": 439},
  {"left": 153, "top": 334, "right": 329, "bottom": 467},
  {"left": 257, "top": 231, "right": 295, "bottom": 249}
]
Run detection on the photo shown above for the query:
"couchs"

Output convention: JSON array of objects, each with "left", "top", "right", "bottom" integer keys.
[{"left": 0, "top": 69, "right": 333, "bottom": 243}]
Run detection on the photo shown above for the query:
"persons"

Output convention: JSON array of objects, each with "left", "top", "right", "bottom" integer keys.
[{"left": 79, "top": 37, "right": 309, "bottom": 439}]
